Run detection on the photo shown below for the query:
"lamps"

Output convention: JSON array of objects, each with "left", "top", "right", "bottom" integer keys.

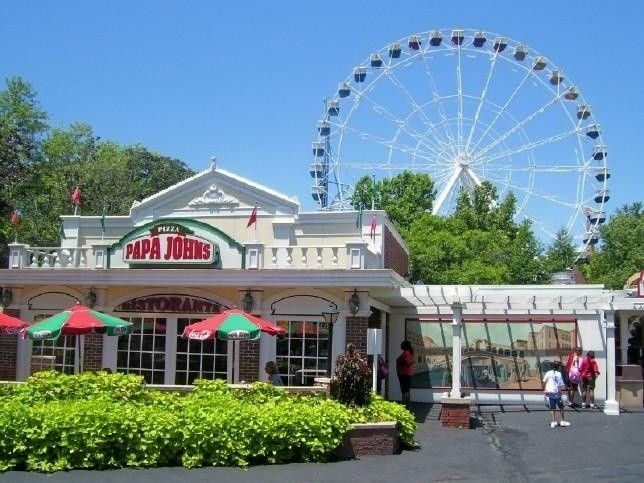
[
  {"left": 85, "top": 285, "right": 97, "bottom": 308},
  {"left": 349, "top": 288, "right": 360, "bottom": 316},
  {"left": 2, "top": 288, "right": 13, "bottom": 308},
  {"left": 240, "top": 288, "right": 254, "bottom": 313}
]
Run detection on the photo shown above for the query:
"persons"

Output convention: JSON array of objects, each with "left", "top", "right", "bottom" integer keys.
[
  {"left": 264, "top": 360, "right": 284, "bottom": 386},
  {"left": 576, "top": 351, "right": 600, "bottom": 409},
  {"left": 395, "top": 340, "right": 415, "bottom": 403},
  {"left": 567, "top": 346, "right": 583, "bottom": 406},
  {"left": 542, "top": 360, "right": 570, "bottom": 428}
]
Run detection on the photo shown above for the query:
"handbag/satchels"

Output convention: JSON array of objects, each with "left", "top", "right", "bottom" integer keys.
[
  {"left": 544, "top": 379, "right": 561, "bottom": 399},
  {"left": 569, "top": 365, "right": 581, "bottom": 384}
]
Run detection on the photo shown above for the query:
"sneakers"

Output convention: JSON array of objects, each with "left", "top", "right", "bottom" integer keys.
[
  {"left": 550, "top": 420, "right": 571, "bottom": 427},
  {"left": 567, "top": 400, "right": 597, "bottom": 408}
]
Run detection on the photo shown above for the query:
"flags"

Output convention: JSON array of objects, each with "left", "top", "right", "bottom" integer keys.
[
  {"left": 245, "top": 205, "right": 256, "bottom": 228},
  {"left": 100, "top": 208, "right": 105, "bottom": 232},
  {"left": 369, "top": 215, "right": 376, "bottom": 238},
  {"left": 355, "top": 206, "right": 362, "bottom": 228}
]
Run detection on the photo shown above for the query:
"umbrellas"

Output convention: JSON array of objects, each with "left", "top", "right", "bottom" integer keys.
[
  {"left": 0, "top": 311, "right": 29, "bottom": 335},
  {"left": 18, "top": 298, "right": 134, "bottom": 372},
  {"left": 178, "top": 304, "right": 288, "bottom": 382}
]
